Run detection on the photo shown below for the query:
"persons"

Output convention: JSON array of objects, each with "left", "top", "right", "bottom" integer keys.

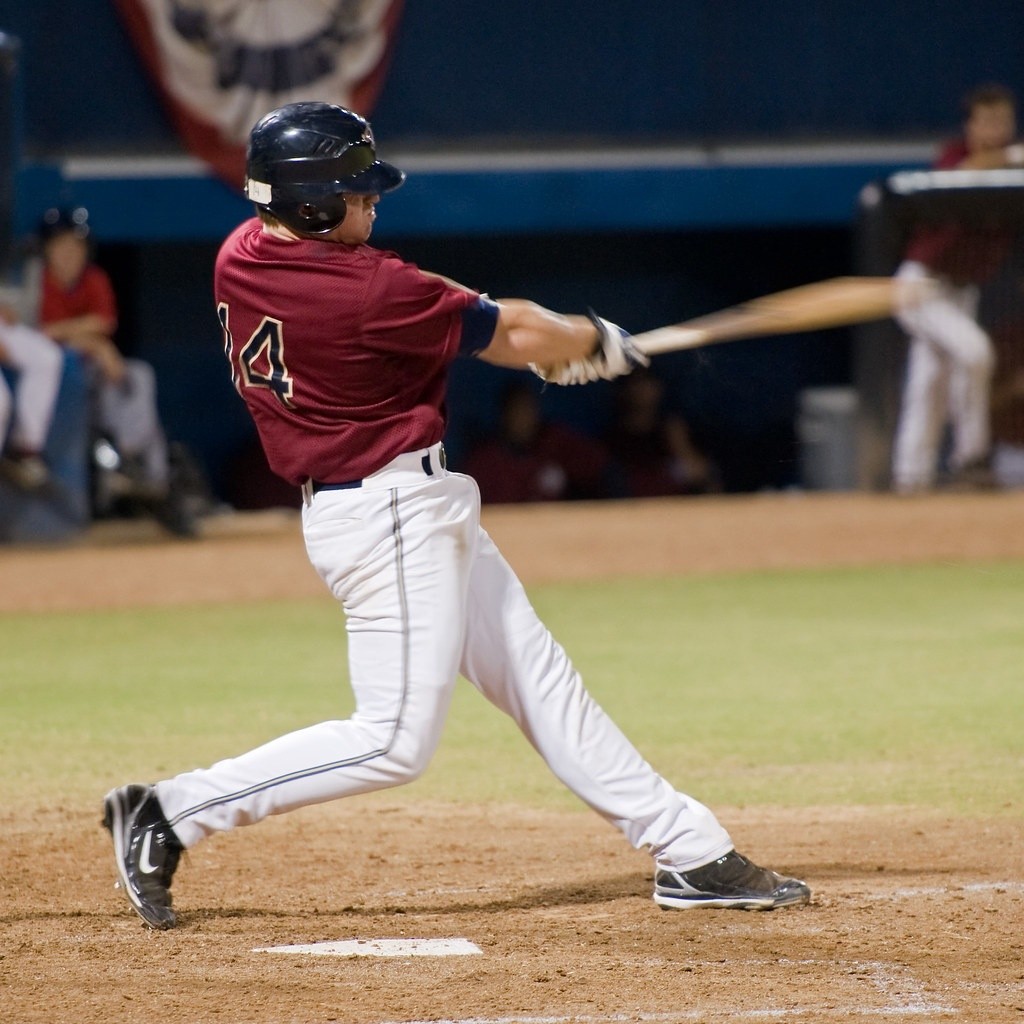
[
  {"left": 885, "top": 82, "right": 1024, "bottom": 495},
  {"left": 0, "top": 212, "right": 170, "bottom": 497},
  {"left": 462, "top": 364, "right": 730, "bottom": 500},
  {"left": 103, "top": 101, "right": 814, "bottom": 927}
]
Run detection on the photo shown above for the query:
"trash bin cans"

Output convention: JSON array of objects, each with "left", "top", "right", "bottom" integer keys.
[{"left": 795, "top": 386, "right": 875, "bottom": 490}]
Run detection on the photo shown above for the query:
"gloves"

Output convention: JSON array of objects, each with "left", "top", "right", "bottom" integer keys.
[
  {"left": 528, "top": 357, "right": 598, "bottom": 386},
  {"left": 588, "top": 305, "right": 652, "bottom": 379}
]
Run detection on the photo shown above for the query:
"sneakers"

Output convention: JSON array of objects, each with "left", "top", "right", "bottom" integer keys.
[
  {"left": 104, "top": 785, "right": 185, "bottom": 929},
  {"left": 652, "top": 851, "right": 811, "bottom": 910}
]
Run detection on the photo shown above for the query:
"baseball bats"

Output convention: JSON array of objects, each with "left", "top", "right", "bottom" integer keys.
[{"left": 539, "top": 275, "right": 934, "bottom": 381}]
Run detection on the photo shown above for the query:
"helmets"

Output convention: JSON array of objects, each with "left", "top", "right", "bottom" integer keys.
[{"left": 242, "top": 101, "right": 406, "bottom": 234}]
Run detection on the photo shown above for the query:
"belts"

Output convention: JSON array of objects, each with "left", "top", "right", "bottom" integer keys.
[{"left": 312, "top": 447, "right": 446, "bottom": 491}]
